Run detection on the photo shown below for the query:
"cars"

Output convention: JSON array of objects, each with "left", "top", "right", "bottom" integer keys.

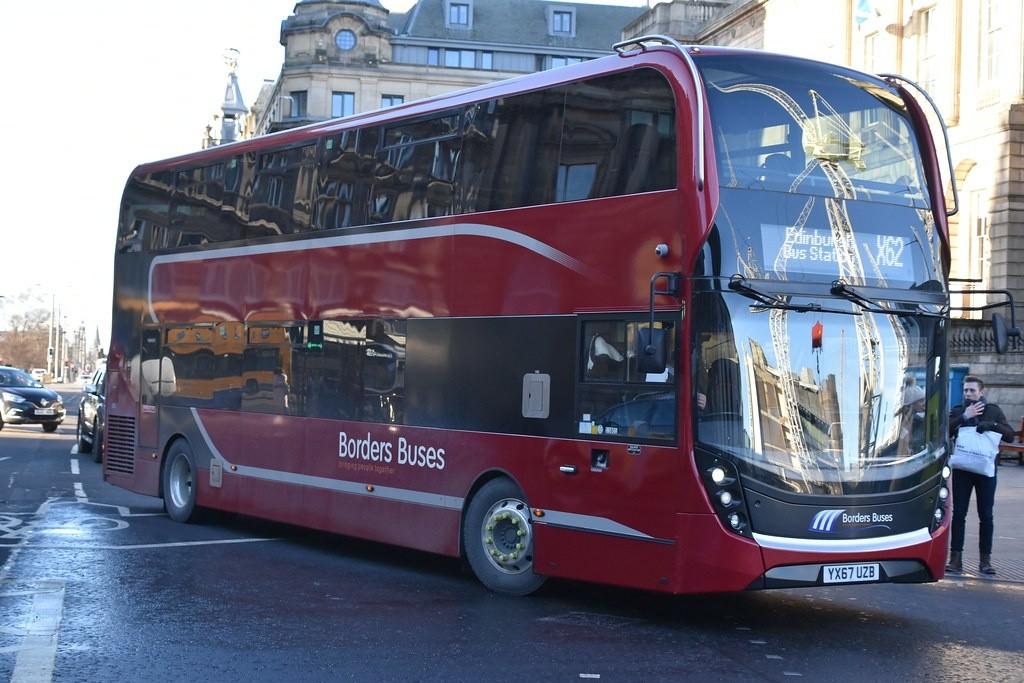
[
  {"left": 18, "top": 366, "right": 98, "bottom": 386},
  {"left": 77, "top": 360, "right": 105, "bottom": 463},
  {"left": 0, "top": 365, "right": 67, "bottom": 433}
]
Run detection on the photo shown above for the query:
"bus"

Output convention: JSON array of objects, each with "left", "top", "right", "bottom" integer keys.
[{"left": 103, "top": 32, "right": 1024, "bottom": 601}]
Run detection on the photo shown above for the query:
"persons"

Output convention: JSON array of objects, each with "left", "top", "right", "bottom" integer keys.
[
  {"left": 897, "top": 375, "right": 926, "bottom": 453},
  {"left": 946, "top": 376, "right": 1016, "bottom": 574},
  {"left": 615, "top": 323, "right": 711, "bottom": 416},
  {"left": 272, "top": 367, "right": 326, "bottom": 417}
]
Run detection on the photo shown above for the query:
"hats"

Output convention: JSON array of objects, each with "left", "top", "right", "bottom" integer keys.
[{"left": 901, "top": 376, "right": 925, "bottom": 405}]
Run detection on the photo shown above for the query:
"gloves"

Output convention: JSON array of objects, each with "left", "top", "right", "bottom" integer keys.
[{"left": 976, "top": 421, "right": 996, "bottom": 434}]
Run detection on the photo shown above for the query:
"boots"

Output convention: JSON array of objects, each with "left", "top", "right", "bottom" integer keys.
[
  {"left": 947, "top": 552, "right": 963, "bottom": 572},
  {"left": 979, "top": 553, "right": 997, "bottom": 574}
]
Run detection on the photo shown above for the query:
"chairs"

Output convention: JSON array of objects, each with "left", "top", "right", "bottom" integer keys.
[{"left": 582, "top": 333, "right": 627, "bottom": 428}]
